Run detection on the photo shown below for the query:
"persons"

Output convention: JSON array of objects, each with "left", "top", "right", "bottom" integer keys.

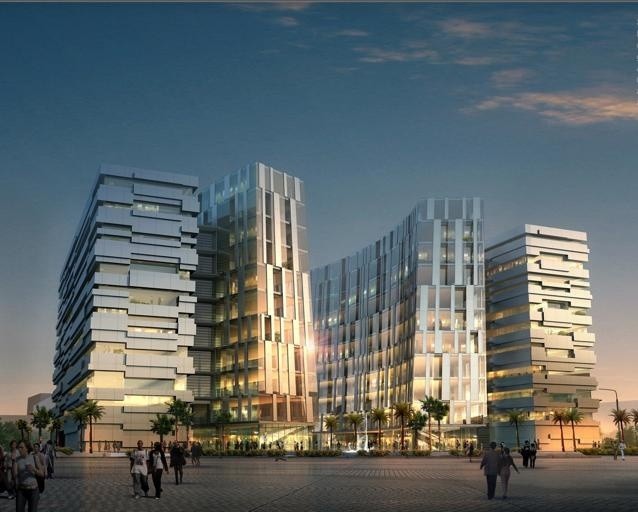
[
  {"left": 592, "top": 439, "right": 626, "bottom": 461},
  {"left": 97, "top": 435, "right": 203, "bottom": 500},
  {"left": 499, "top": 447, "right": 521, "bottom": 499},
  {"left": 500, "top": 442, "right": 505, "bottom": 448},
  {"left": 205, "top": 438, "right": 410, "bottom": 451},
  {"left": 521, "top": 440, "right": 538, "bottom": 469},
  {"left": 479, "top": 442, "right": 499, "bottom": 500},
  {"left": 499, "top": 443, "right": 504, "bottom": 457},
  {"left": 454, "top": 439, "right": 474, "bottom": 463},
  {"left": 0, "top": 433, "right": 58, "bottom": 512}
]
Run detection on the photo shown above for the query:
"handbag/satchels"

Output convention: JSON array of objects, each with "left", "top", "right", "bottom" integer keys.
[
  {"left": 148, "top": 465, "right": 157, "bottom": 474},
  {"left": 33, "top": 455, "right": 46, "bottom": 493}
]
